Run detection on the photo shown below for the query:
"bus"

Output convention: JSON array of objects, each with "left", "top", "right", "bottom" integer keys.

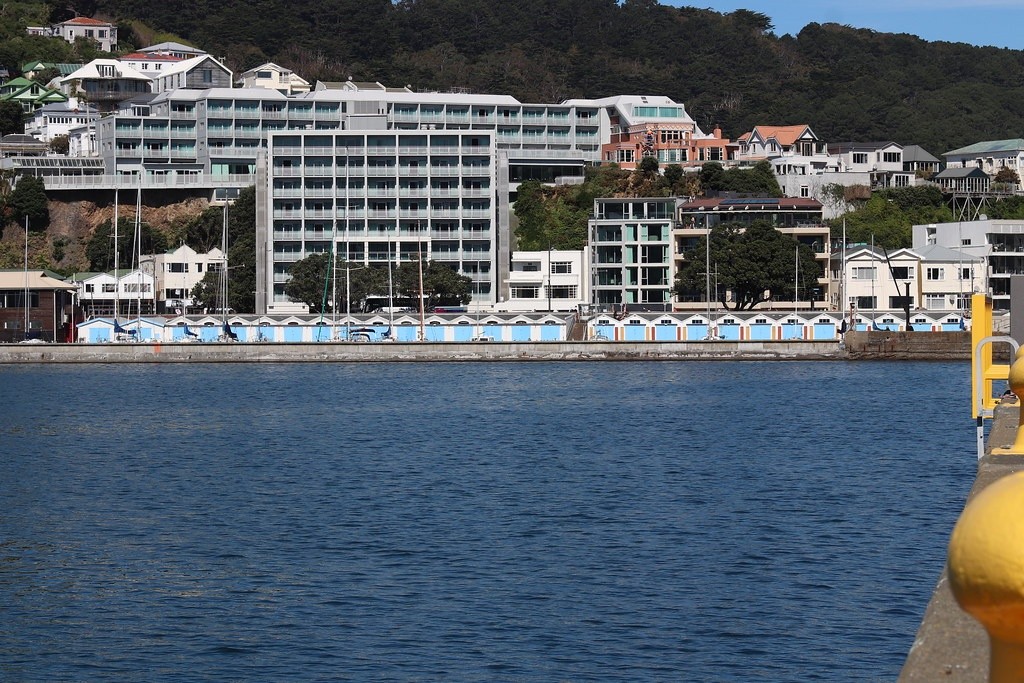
[{"left": 360, "top": 293, "right": 469, "bottom": 315}]
